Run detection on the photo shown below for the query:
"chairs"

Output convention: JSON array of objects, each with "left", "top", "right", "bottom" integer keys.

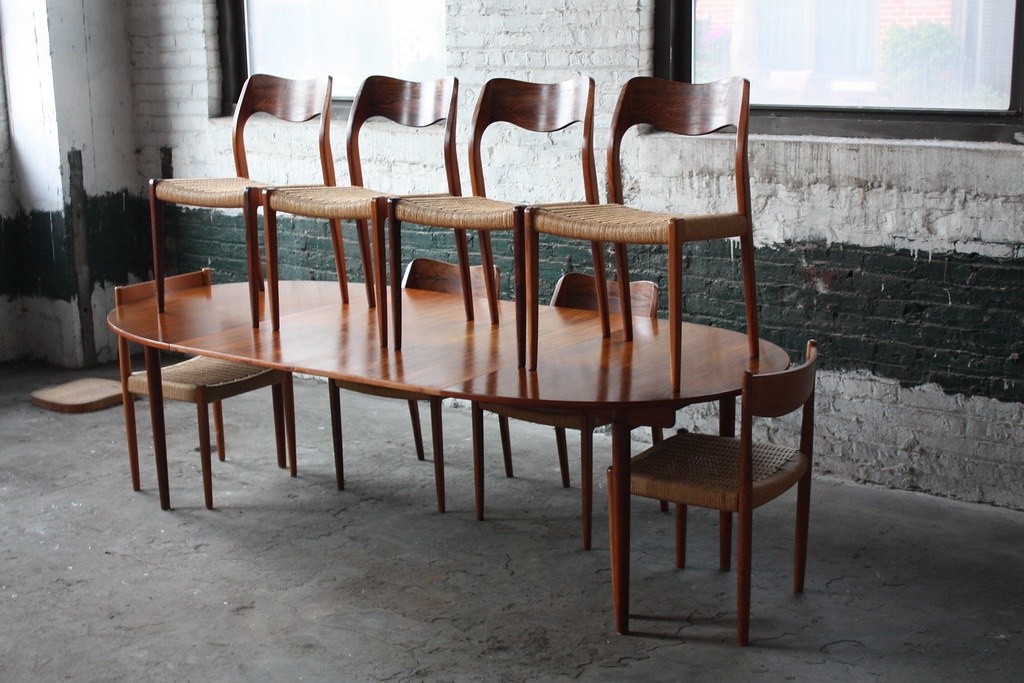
[
  {"left": 114, "top": 267, "right": 287, "bottom": 510},
  {"left": 471, "top": 272, "right": 669, "bottom": 551},
  {"left": 148, "top": 74, "right": 349, "bottom": 327},
  {"left": 329, "top": 259, "right": 514, "bottom": 514},
  {"left": 524, "top": 76, "right": 759, "bottom": 393},
  {"left": 262, "top": 75, "right": 474, "bottom": 348},
  {"left": 606, "top": 338, "right": 818, "bottom": 645},
  {"left": 388, "top": 78, "right": 611, "bottom": 367}
]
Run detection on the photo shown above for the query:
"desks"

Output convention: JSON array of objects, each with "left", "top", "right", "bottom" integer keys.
[{"left": 106, "top": 280, "right": 791, "bottom": 635}]
